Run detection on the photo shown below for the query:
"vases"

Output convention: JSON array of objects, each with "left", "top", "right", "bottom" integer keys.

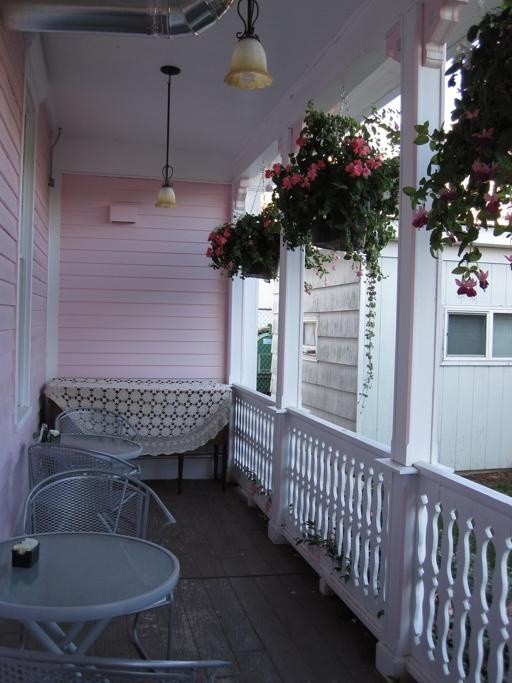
[
  {"left": 245, "top": 263, "right": 265, "bottom": 278},
  {"left": 312, "top": 216, "right": 364, "bottom": 250}
]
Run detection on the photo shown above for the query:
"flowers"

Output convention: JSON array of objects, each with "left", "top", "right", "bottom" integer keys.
[
  {"left": 265, "top": 84, "right": 401, "bottom": 420},
  {"left": 206, "top": 208, "right": 279, "bottom": 283},
  {"left": 401, "top": 0, "right": 512, "bottom": 297}
]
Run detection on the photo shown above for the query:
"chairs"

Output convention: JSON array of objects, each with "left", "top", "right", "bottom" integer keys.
[
  {"left": 3, "top": 647, "right": 233, "bottom": 681},
  {"left": 55, "top": 408, "right": 142, "bottom": 532},
  {"left": 29, "top": 443, "right": 144, "bottom": 534},
  {"left": 24, "top": 469, "right": 178, "bottom": 674}
]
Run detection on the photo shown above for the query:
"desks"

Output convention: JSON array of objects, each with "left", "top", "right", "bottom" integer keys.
[
  {"left": 58, "top": 433, "right": 142, "bottom": 534},
  {"left": 1, "top": 531, "right": 181, "bottom": 682},
  {"left": 44, "top": 376, "right": 234, "bottom": 494}
]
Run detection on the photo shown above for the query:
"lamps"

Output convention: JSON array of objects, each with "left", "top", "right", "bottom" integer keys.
[
  {"left": 154, "top": 66, "right": 181, "bottom": 207},
  {"left": 224, "top": 0, "right": 273, "bottom": 91}
]
